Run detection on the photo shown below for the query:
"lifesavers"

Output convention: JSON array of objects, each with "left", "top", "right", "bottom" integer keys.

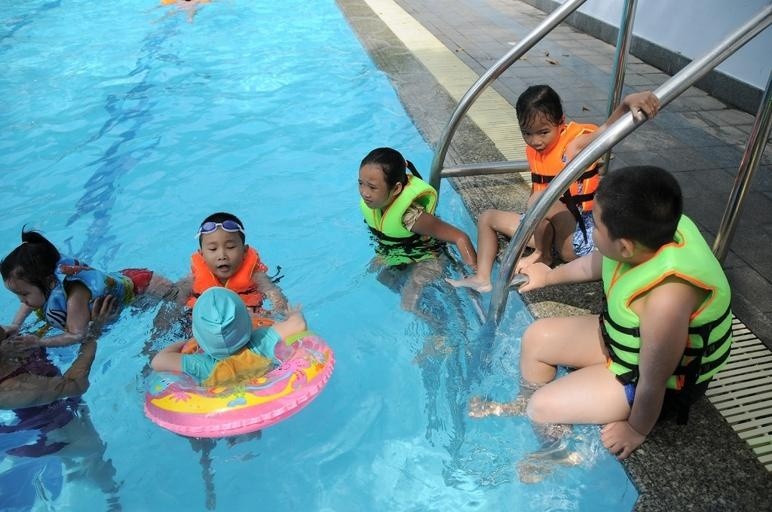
[{"left": 143, "top": 318, "right": 336, "bottom": 438}]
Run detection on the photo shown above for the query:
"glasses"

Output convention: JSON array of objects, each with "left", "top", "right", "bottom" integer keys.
[{"left": 198, "top": 220, "right": 245, "bottom": 235}]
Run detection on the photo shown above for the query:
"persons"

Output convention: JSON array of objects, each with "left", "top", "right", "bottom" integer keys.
[
  {"left": 443, "top": 84, "right": 661, "bottom": 294},
  {"left": 0, "top": 221, "right": 176, "bottom": 346},
  {"left": 468, "top": 164, "right": 735, "bottom": 487},
  {"left": 0, "top": 292, "right": 127, "bottom": 460},
  {"left": 139, "top": 211, "right": 288, "bottom": 381},
  {"left": 355, "top": 146, "right": 475, "bottom": 368},
  {"left": 148, "top": 285, "right": 309, "bottom": 391}
]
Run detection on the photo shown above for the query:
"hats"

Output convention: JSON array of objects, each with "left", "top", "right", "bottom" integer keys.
[{"left": 191, "top": 286, "right": 252, "bottom": 360}]
